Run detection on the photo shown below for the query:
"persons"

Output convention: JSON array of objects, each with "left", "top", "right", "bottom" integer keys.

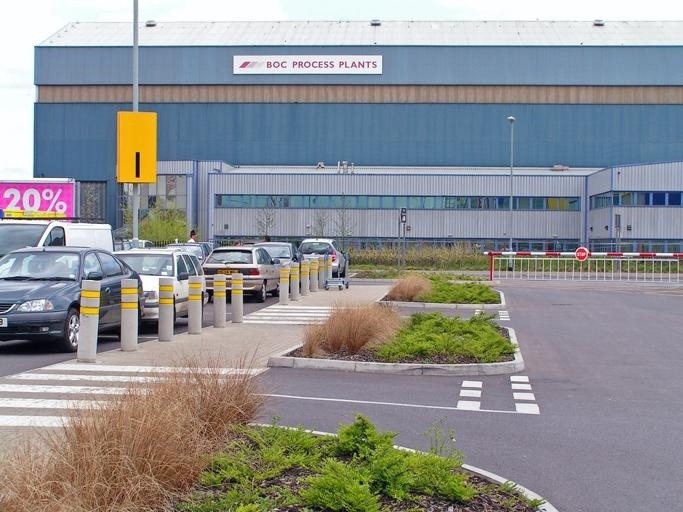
[
  {"left": 263, "top": 236, "right": 271, "bottom": 242},
  {"left": 186, "top": 230, "right": 197, "bottom": 242}
]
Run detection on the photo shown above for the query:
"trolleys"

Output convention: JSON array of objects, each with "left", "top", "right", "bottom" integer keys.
[{"left": 324, "top": 249, "right": 349, "bottom": 291}]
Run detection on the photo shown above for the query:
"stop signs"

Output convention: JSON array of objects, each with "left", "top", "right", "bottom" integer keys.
[{"left": 575, "top": 246, "right": 589, "bottom": 262}]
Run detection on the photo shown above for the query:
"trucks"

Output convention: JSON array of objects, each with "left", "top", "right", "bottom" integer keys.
[{"left": 0, "top": 218, "right": 114, "bottom": 267}]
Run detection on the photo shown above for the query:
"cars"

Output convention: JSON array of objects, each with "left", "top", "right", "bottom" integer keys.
[
  {"left": 298, "top": 238, "right": 349, "bottom": 278},
  {"left": 202, "top": 246, "right": 280, "bottom": 304},
  {"left": 0, "top": 245, "right": 146, "bottom": 353},
  {"left": 253, "top": 242, "right": 305, "bottom": 293},
  {"left": 113, "top": 237, "right": 213, "bottom": 266},
  {"left": 111, "top": 248, "right": 210, "bottom": 323}
]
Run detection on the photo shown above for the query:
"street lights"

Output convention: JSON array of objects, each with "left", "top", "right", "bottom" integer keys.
[
  {"left": 131, "top": 0, "right": 157, "bottom": 239},
  {"left": 507, "top": 115, "right": 516, "bottom": 271}
]
